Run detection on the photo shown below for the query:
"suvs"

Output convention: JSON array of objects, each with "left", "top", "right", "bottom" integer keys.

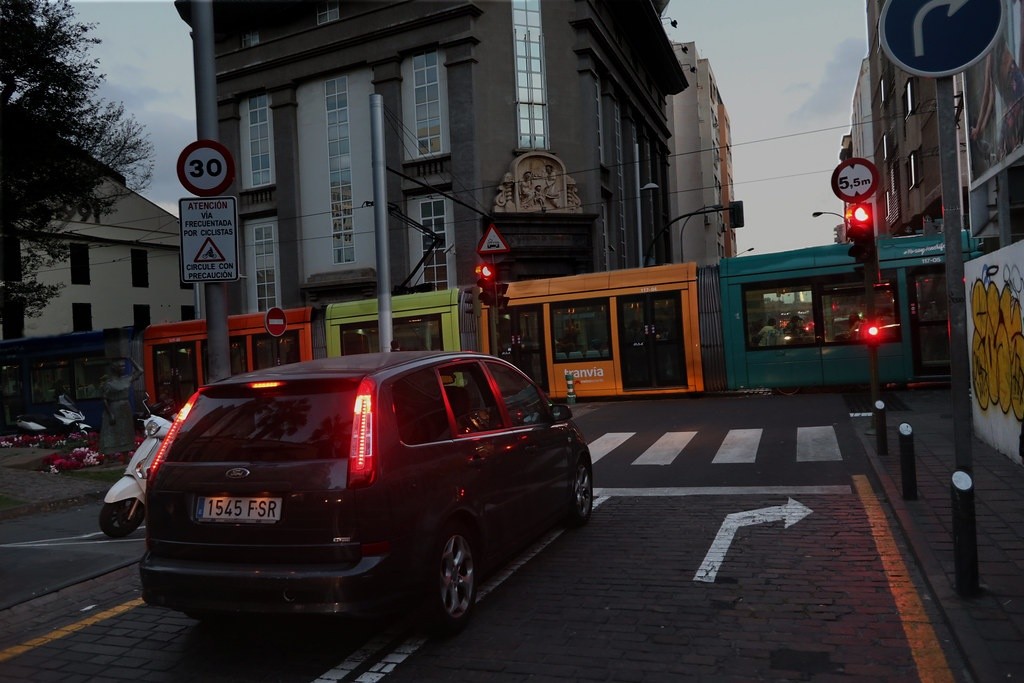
[{"left": 138, "top": 349, "right": 596, "bottom": 638}]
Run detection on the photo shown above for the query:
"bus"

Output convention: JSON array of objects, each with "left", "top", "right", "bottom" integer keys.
[{"left": 0, "top": 229, "right": 984, "bottom": 436}]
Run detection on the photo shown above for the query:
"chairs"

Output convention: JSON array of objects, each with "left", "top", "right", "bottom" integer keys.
[
  {"left": 556, "top": 349, "right": 609, "bottom": 359},
  {"left": 432, "top": 386, "right": 477, "bottom": 433}
]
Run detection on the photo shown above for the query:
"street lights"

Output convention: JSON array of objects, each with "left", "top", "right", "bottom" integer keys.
[
  {"left": 812, "top": 209, "right": 880, "bottom": 431},
  {"left": 680, "top": 203, "right": 723, "bottom": 267}
]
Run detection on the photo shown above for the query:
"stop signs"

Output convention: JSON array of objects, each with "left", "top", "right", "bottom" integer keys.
[{"left": 264, "top": 307, "right": 289, "bottom": 337}]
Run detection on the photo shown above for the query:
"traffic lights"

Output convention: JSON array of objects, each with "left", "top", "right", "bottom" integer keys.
[
  {"left": 864, "top": 322, "right": 884, "bottom": 346},
  {"left": 476, "top": 264, "right": 496, "bottom": 308},
  {"left": 495, "top": 282, "right": 511, "bottom": 310},
  {"left": 847, "top": 203, "right": 880, "bottom": 267},
  {"left": 463, "top": 285, "right": 478, "bottom": 316}
]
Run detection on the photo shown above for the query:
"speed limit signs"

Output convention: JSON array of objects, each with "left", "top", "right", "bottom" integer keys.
[{"left": 176, "top": 138, "right": 236, "bottom": 199}]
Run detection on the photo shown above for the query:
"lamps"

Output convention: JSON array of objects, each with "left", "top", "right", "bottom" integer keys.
[
  {"left": 681, "top": 63, "right": 696, "bottom": 74},
  {"left": 672, "top": 44, "right": 688, "bottom": 54},
  {"left": 660, "top": 17, "right": 677, "bottom": 29}
]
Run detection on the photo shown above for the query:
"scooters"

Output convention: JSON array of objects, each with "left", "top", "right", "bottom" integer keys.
[
  {"left": 98, "top": 391, "right": 181, "bottom": 537},
  {"left": 16, "top": 394, "right": 93, "bottom": 437}
]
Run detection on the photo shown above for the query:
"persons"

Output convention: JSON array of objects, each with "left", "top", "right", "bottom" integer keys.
[
  {"left": 446, "top": 385, "right": 472, "bottom": 418},
  {"left": 591, "top": 338, "right": 603, "bottom": 350},
  {"left": 629, "top": 320, "right": 648, "bottom": 352},
  {"left": 101, "top": 355, "right": 144, "bottom": 466},
  {"left": 390, "top": 340, "right": 401, "bottom": 352},
  {"left": 849, "top": 311, "right": 860, "bottom": 328},
  {"left": 756, "top": 318, "right": 777, "bottom": 346},
  {"left": 518, "top": 171, "right": 534, "bottom": 207},
  {"left": 554, "top": 338, "right": 566, "bottom": 348},
  {"left": 544, "top": 165, "right": 560, "bottom": 208},
  {"left": 783, "top": 316, "right": 809, "bottom": 344},
  {"left": 969, "top": 30, "right": 1024, "bottom": 159},
  {"left": 534, "top": 185, "right": 546, "bottom": 208}
]
